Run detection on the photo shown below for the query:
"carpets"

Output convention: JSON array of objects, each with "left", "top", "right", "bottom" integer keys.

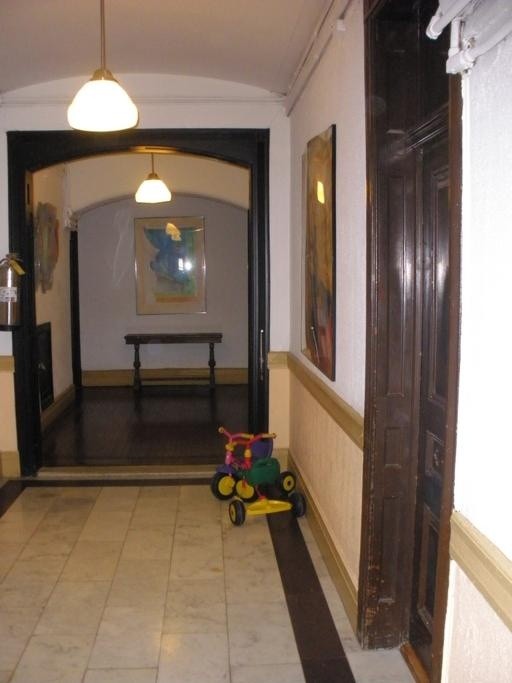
[{"left": 38, "top": 385, "right": 250, "bottom": 465}]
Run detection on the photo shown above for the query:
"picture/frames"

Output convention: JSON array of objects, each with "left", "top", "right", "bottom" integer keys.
[{"left": 133, "top": 216, "right": 206, "bottom": 315}]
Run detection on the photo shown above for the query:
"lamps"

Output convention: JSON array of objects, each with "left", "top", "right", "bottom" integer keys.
[
  {"left": 68, "top": 0, "right": 138, "bottom": 134},
  {"left": 135, "top": 153, "right": 171, "bottom": 203}
]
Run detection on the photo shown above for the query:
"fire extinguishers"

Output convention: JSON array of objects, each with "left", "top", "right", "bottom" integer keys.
[{"left": 1, "top": 253, "right": 25, "bottom": 330}]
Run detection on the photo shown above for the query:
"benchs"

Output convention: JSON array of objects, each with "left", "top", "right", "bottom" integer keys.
[{"left": 125, "top": 332, "right": 222, "bottom": 410}]
